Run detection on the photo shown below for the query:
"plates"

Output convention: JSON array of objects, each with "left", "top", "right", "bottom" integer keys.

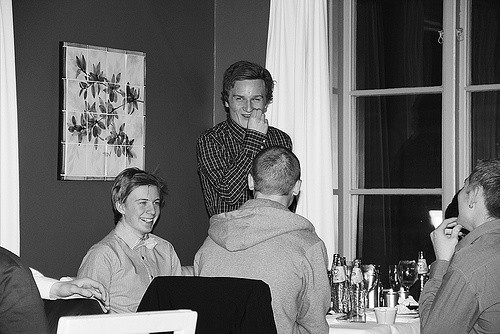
[{"left": 396, "top": 314, "right": 419, "bottom": 319}]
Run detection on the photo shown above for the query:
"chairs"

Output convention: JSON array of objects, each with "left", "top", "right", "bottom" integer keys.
[
  {"left": 42, "top": 299, "right": 104, "bottom": 334},
  {"left": 136, "top": 276, "right": 278, "bottom": 334},
  {"left": 56, "top": 309, "right": 198, "bottom": 334}
]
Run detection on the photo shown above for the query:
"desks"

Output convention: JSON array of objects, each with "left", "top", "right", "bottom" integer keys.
[{"left": 326, "top": 308, "right": 421, "bottom": 334}]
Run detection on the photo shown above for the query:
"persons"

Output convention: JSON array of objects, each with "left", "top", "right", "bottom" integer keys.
[
  {"left": 77, "top": 168, "right": 181, "bottom": 314},
  {"left": 28, "top": 267, "right": 111, "bottom": 312},
  {"left": 192, "top": 146, "right": 331, "bottom": 334},
  {"left": 420, "top": 161, "right": 500, "bottom": 334},
  {"left": 197, "top": 61, "right": 298, "bottom": 216},
  {"left": 0, "top": 246, "right": 49, "bottom": 334}
]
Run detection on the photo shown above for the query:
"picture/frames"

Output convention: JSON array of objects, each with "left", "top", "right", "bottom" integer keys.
[{"left": 56, "top": 41, "right": 147, "bottom": 182}]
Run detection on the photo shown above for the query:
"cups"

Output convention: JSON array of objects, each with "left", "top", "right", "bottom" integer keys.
[
  {"left": 388, "top": 264, "right": 398, "bottom": 291},
  {"left": 360, "top": 265, "right": 380, "bottom": 309}
]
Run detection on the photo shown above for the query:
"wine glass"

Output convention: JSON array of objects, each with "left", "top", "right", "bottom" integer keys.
[{"left": 397, "top": 260, "right": 418, "bottom": 300}]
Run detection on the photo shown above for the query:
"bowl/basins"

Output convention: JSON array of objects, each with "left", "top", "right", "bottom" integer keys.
[
  {"left": 373, "top": 307, "right": 398, "bottom": 324},
  {"left": 406, "top": 306, "right": 419, "bottom": 310}
]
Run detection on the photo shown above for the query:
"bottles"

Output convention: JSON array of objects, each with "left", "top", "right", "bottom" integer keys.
[
  {"left": 327, "top": 254, "right": 366, "bottom": 323},
  {"left": 416, "top": 251, "right": 430, "bottom": 292}
]
